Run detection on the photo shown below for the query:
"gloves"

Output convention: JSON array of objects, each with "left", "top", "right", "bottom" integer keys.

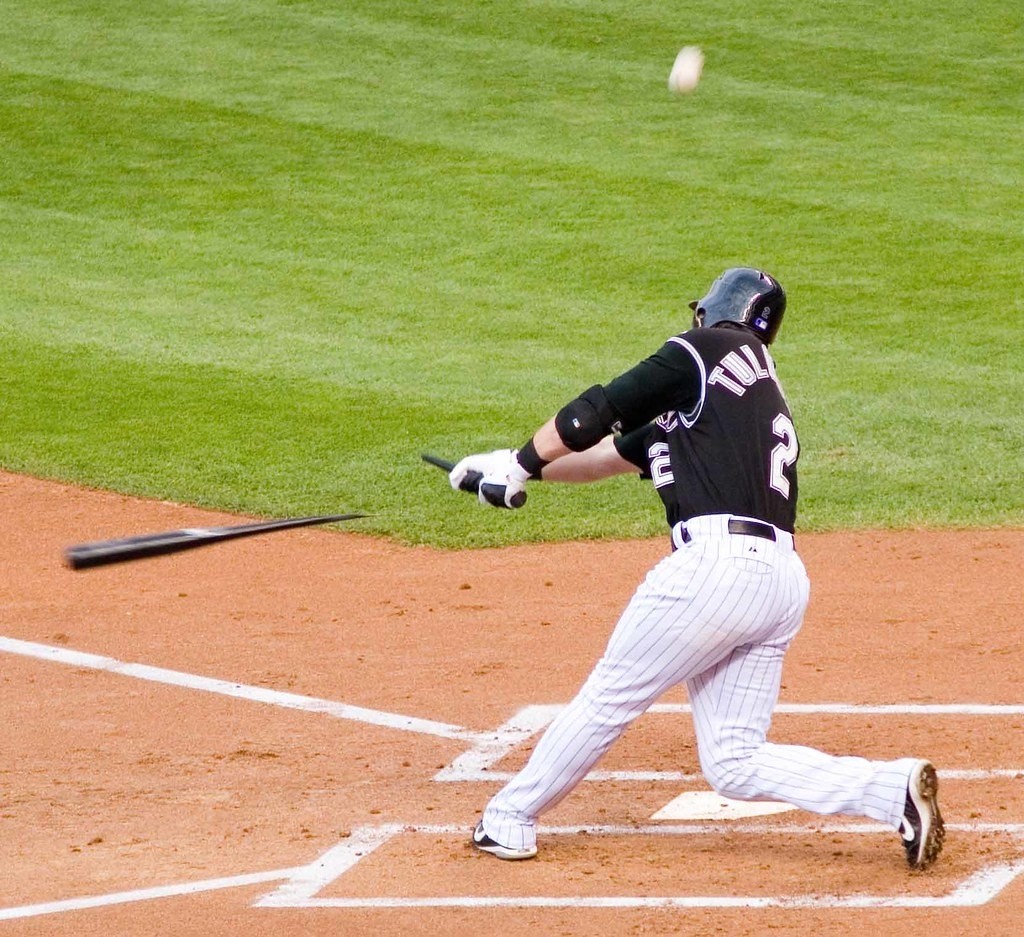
[
  {"left": 478, "top": 439, "right": 552, "bottom": 507},
  {"left": 449, "top": 448, "right": 542, "bottom": 492}
]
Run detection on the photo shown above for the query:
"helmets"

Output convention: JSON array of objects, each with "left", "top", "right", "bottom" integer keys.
[{"left": 689, "top": 268, "right": 786, "bottom": 344}]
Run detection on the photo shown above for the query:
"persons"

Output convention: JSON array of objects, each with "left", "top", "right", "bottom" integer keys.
[{"left": 448, "top": 267, "right": 946, "bottom": 871}]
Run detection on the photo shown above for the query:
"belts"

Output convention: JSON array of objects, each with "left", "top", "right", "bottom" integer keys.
[{"left": 671, "top": 519, "right": 795, "bottom": 553}]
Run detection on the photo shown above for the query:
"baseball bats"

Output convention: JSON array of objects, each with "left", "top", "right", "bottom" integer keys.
[
  {"left": 415, "top": 451, "right": 529, "bottom": 510},
  {"left": 62, "top": 510, "right": 371, "bottom": 572}
]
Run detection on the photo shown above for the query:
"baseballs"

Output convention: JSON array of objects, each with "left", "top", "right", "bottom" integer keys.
[{"left": 668, "top": 42, "right": 708, "bottom": 92}]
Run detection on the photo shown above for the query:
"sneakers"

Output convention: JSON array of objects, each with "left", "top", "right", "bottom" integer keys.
[
  {"left": 897, "top": 759, "right": 946, "bottom": 871},
  {"left": 472, "top": 820, "right": 537, "bottom": 860}
]
